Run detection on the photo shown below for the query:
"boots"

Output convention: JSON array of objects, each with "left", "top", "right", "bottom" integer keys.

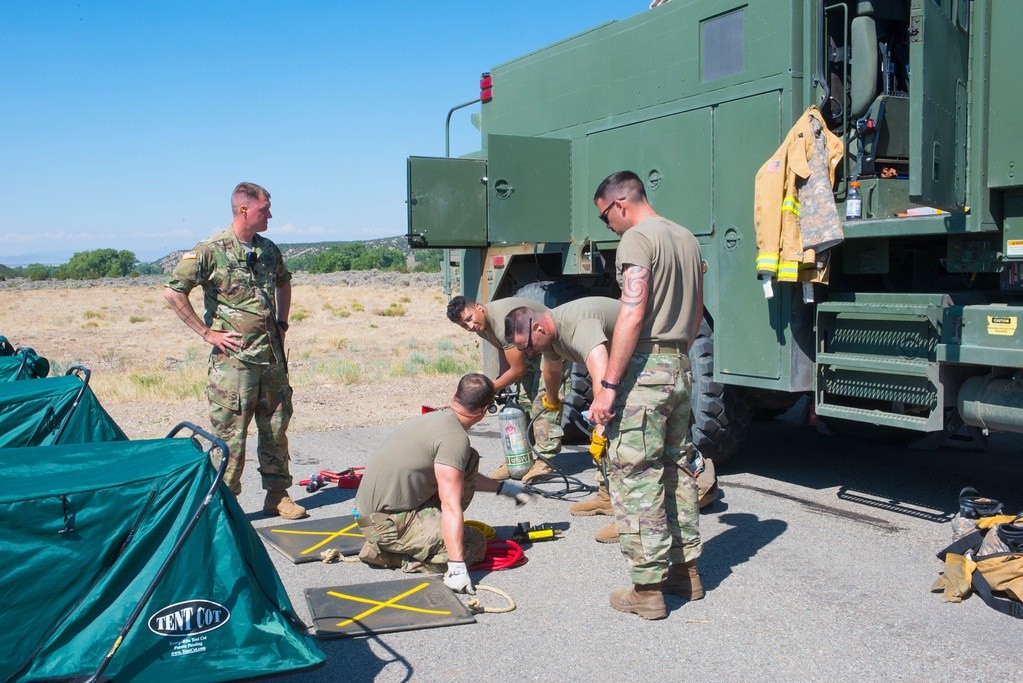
[
  {"left": 568, "top": 481, "right": 614, "bottom": 517},
  {"left": 662, "top": 557, "right": 704, "bottom": 601},
  {"left": 596, "top": 522, "right": 620, "bottom": 543},
  {"left": 263, "top": 489, "right": 306, "bottom": 519},
  {"left": 610, "top": 583, "right": 666, "bottom": 621}
]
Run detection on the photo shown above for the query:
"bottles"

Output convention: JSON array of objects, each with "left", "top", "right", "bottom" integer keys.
[{"left": 845, "top": 182, "right": 861, "bottom": 220}]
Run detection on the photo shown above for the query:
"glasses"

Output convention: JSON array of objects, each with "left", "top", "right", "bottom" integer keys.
[
  {"left": 599, "top": 195, "right": 626, "bottom": 224},
  {"left": 517, "top": 317, "right": 534, "bottom": 354}
]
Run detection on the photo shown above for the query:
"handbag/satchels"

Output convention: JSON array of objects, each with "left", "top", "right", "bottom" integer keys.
[
  {"left": 952, "top": 486, "right": 1005, "bottom": 542},
  {"left": 682, "top": 443, "right": 719, "bottom": 508},
  {"left": 965, "top": 524, "right": 1023, "bottom": 619}
]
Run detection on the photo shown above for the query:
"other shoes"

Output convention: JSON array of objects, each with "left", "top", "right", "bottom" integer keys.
[
  {"left": 997, "top": 523, "right": 1023, "bottom": 553},
  {"left": 359, "top": 541, "right": 405, "bottom": 568},
  {"left": 403, "top": 555, "right": 447, "bottom": 573}
]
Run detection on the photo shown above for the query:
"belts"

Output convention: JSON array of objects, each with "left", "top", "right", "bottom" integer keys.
[
  {"left": 636, "top": 339, "right": 687, "bottom": 355},
  {"left": 355, "top": 511, "right": 392, "bottom": 528}
]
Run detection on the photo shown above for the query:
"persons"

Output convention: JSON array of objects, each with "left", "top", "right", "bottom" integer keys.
[
  {"left": 161, "top": 182, "right": 306, "bottom": 518},
  {"left": 354, "top": 372, "right": 533, "bottom": 596},
  {"left": 588, "top": 170, "right": 705, "bottom": 619},
  {"left": 500, "top": 295, "right": 621, "bottom": 544},
  {"left": 447, "top": 296, "right": 574, "bottom": 482}
]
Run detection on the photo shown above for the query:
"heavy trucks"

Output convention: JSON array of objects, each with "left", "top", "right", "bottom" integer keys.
[{"left": 404, "top": 1, "right": 1023, "bottom": 469}]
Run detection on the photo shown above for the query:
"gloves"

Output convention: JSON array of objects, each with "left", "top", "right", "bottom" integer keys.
[
  {"left": 973, "top": 512, "right": 1023, "bottom": 531},
  {"left": 931, "top": 550, "right": 976, "bottom": 603},
  {"left": 443, "top": 561, "right": 476, "bottom": 595},
  {"left": 542, "top": 393, "right": 562, "bottom": 411},
  {"left": 590, "top": 430, "right": 608, "bottom": 465},
  {"left": 496, "top": 480, "right": 534, "bottom": 505}
]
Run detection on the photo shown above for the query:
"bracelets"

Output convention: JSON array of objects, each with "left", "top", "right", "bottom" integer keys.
[
  {"left": 277, "top": 320, "right": 289, "bottom": 330},
  {"left": 601, "top": 380, "right": 618, "bottom": 389}
]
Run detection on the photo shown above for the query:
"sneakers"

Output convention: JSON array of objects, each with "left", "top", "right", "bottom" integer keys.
[
  {"left": 489, "top": 462, "right": 512, "bottom": 479},
  {"left": 521, "top": 459, "right": 553, "bottom": 482}
]
